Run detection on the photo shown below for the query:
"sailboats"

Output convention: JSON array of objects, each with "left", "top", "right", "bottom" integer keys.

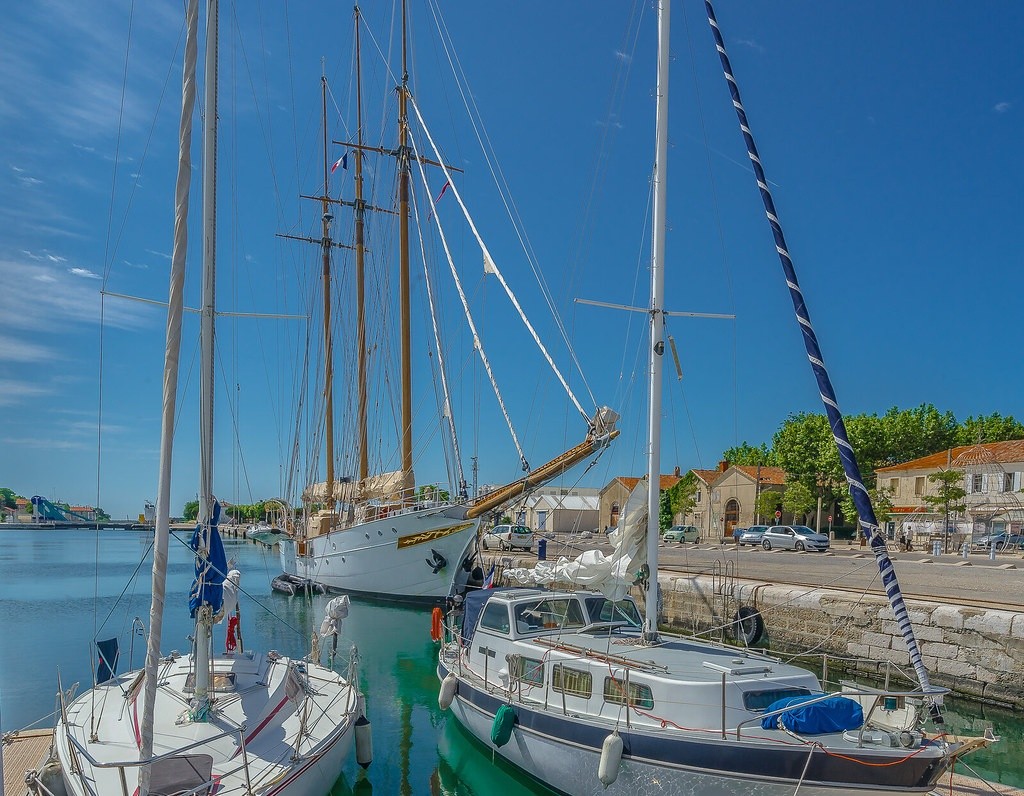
[
  {"left": 46, "top": 0, "right": 370, "bottom": 795},
  {"left": 432, "top": 1, "right": 1007, "bottom": 796},
  {"left": 280, "top": 0, "right": 626, "bottom": 601}
]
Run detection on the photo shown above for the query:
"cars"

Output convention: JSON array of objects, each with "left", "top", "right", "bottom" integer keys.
[
  {"left": 758, "top": 525, "right": 832, "bottom": 554},
  {"left": 976, "top": 529, "right": 1024, "bottom": 552},
  {"left": 739, "top": 525, "right": 771, "bottom": 547},
  {"left": 663, "top": 525, "right": 701, "bottom": 546},
  {"left": 482, "top": 524, "right": 534, "bottom": 554},
  {"left": 605, "top": 526, "right": 618, "bottom": 538}
]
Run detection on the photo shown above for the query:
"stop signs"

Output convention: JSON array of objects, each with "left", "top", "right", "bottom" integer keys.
[
  {"left": 828, "top": 516, "right": 832, "bottom": 522},
  {"left": 775, "top": 511, "right": 781, "bottom": 518}
]
